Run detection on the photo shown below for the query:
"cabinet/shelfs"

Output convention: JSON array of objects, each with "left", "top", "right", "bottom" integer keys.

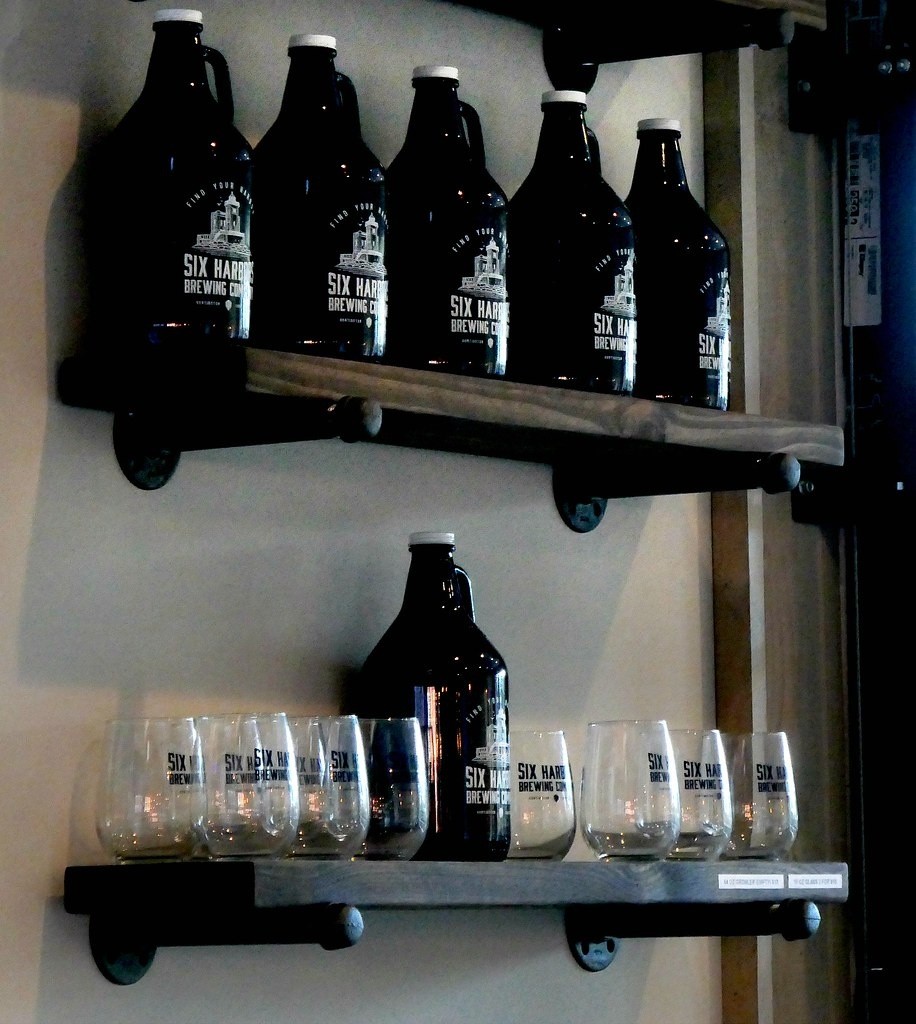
[{"left": 57, "top": 0, "right": 850, "bottom": 986}]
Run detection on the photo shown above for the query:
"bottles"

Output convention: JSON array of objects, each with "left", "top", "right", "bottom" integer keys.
[
  {"left": 250, "top": 32, "right": 392, "bottom": 366},
  {"left": 350, "top": 530, "right": 513, "bottom": 862},
  {"left": 617, "top": 115, "right": 735, "bottom": 414},
  {"left": 89, "top": 8, "right": 257, "bottom": 351},
  {"left": 384, "top": 66, "right": 516, "bottom": 382},
  {"left": 512, "top": 90, "right": 643, "bottom": 398}
]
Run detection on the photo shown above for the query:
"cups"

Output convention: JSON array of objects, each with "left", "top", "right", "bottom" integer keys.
[
  {"left": 665, "top": 729, "right": 734, "bottom": 864},
  {"left": 719, "top": 730, "right": 800, "bottom": 861},
  {"left": 579, "top": 718, "right": 681, "bottom": 861},
  {"left": 93, "top": 714, "right": 429, "bottom": 862},
  {"left": 509, "top": 729, "right": 576, "bottom": 861}
]
